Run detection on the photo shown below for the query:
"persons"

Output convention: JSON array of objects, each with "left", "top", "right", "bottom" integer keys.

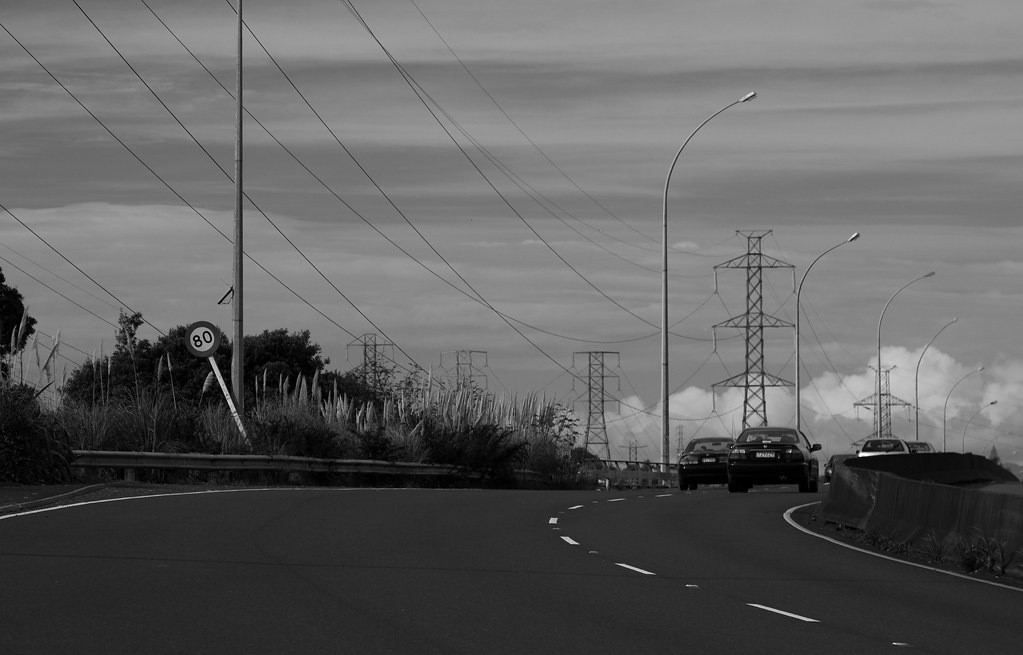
[
  {"left": 701, "top": 445, "right": 707, "bottom": 450},
  {"left": 756, "top": 435, "right": 763, "bottom": 440}
]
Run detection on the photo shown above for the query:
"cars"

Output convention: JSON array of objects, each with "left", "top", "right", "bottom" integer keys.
[
  {"left": 677, "top": 438, "right": 737, "bottom": 492},
  {"left": 907, "top": 441, "right": 935, "bottom": 454},
  {"left": 728, "top": 427, "right": 822, "bottom": 494},
  {"left": 824, "top": 454, "right": 859, "bottom": 481},
  {"left": 856, "top": 438, "right": 909, "bottom": 457}
]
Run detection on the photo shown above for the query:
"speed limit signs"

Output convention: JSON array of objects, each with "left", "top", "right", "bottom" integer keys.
[{"left": 185, "top": 320, "right": 219, "bottom": 358}]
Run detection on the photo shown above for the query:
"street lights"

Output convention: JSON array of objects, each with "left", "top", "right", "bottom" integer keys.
[
  {"left": 795, "top": 232, "right": 860, "bottom": 430},
  {"left": 962, "top": 399, "right": 998, "bottom": 453},
  {"left": 981, "top": 430, "right": 1012, "bottom": 457},
  {"left": 943, "top": 365, "right": 985, "bottom": 452},
  {"left": 915, "top": 316, "right": 959, "bottom": 443},
  {"left": 660, "top": 87, "right": 758, "bottom": 491},
  {"left": 876, "top": 270, "right": 936, "bottom": 438}
]
